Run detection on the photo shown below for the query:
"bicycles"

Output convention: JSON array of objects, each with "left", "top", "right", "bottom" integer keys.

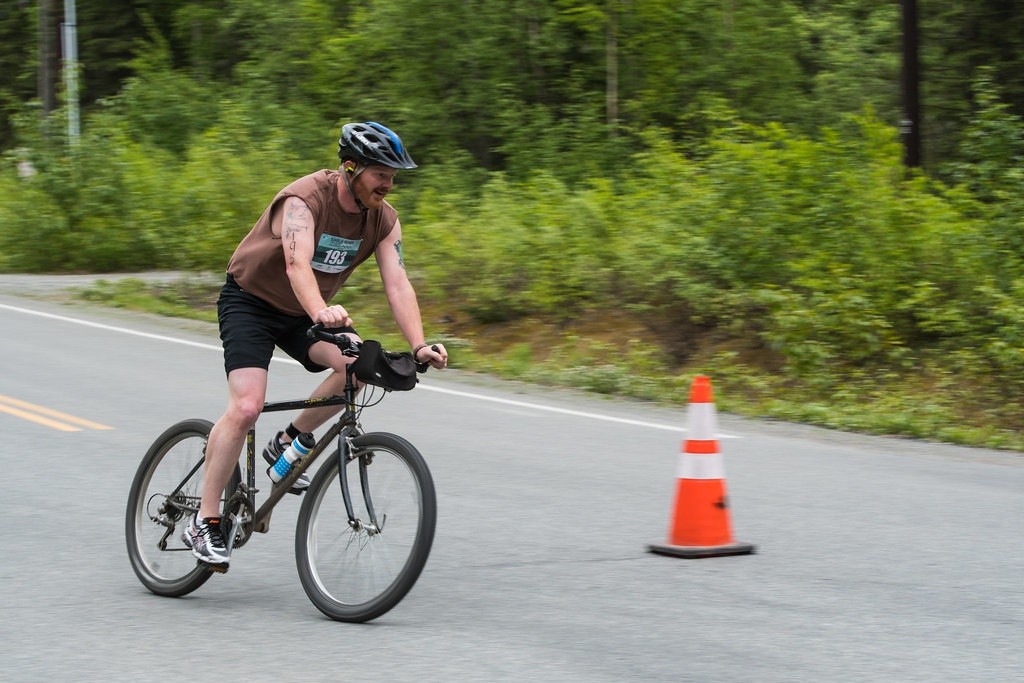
[{"left": 125, "top": 319, "right": 441, "bottom": 625}]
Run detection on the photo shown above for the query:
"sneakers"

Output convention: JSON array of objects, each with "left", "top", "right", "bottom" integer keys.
[
  {"left": 180, "top": 513, "right": 230, "bottom": 569},
  {"left": 263, "top": 430, "right": 310, "bottom": 488}
]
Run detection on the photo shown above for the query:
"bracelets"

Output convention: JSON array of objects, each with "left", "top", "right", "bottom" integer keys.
[{"left": 413, "top": 344, "right": 428, "bottom": 363}]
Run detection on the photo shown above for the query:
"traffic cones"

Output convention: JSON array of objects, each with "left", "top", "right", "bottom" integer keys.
[{"left": 647, "top": 376, "right": 756, "bottom": 559}]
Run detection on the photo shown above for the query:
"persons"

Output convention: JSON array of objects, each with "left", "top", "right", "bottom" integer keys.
[{"left": 181, "top": 120, "right": 449, "bottom": 570}]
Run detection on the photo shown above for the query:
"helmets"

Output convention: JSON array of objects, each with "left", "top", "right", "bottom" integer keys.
[{"left": 338, "top": 123, "right": 418, "bottom": 170}]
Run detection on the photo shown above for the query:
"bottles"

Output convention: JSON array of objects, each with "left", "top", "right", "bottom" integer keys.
[{"left": 268, "top": 432, "right": 315, "bottom": 484}]
